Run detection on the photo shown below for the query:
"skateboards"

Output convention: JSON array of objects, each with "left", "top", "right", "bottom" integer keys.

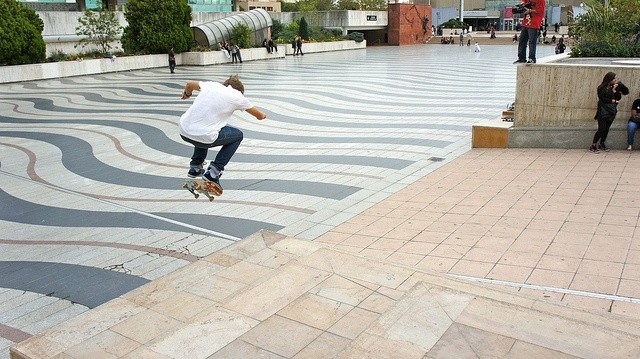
[{"left": 183, "top": 178, "right": 224, "bottom": 201}]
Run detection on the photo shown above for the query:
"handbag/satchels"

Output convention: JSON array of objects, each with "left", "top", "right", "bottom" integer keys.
[{"left": 169, "top": 56, "right": 175, "bottom": 62}]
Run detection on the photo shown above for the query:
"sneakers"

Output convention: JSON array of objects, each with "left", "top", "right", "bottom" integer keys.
[
  {"left": 202, "top": 170, "right": 223, "bottom": 190},
  {"left": 512, "top": 60, "right": 527, "bottom": 64},
  {"left": 187, "top": 168, "right": 205, "bottom": 177},
  {"left": 527, "top": 59, "right": 536, "bottom": 64},
  {"left": 597, "top": 143, "right": 610, "bottom": 152},
  {"left": 589, "top": 144, "right": 600, "bottom": 154},
  {"left": 627, "top": 145, "right": 633, "bottom": 151}
]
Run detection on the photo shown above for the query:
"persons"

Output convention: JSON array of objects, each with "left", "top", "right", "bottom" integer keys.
[
  {"left": 627, "top": 92, "right": 640, "bottom": 151},
  {"left": 168, "top": 47, "right": 176, "bottom": 73},
  {"left": 538, "top": 19, "right": 566, "bottom": 54},
  {"left": 263, "top": 39, "right": 271, "bottom": 53},
  {"left": 269, "top": 39, "right": 277, "bottom": 54},
  {"left": 234, "top": 44, "right": 243, "bottom": 63},
  {"left": 513, "top": 0, "right": 546, "bottom": 64},
  {"left": 589, "top": 72, "right": 629, "bottom": 153},
  {"left": 177, "top": 74, "right": 266, "bottom": 189},
  {"left": 231, "top": 42, "right": 238, "bottom": 63},
  {"left": 291, "top": 37, "right": 297, "bottom": 56},
  {"left": 431, "top": 25, "right": 497, "bottom": 52},
  {"left": 217, "top": 41, "right": 231, "bottom": 59},
  {"left": 224, "top": 40, "right": 230, "bottom": 55},
  {"left": 296, "top": 37, "right": 303, "bottom": 55}
]
